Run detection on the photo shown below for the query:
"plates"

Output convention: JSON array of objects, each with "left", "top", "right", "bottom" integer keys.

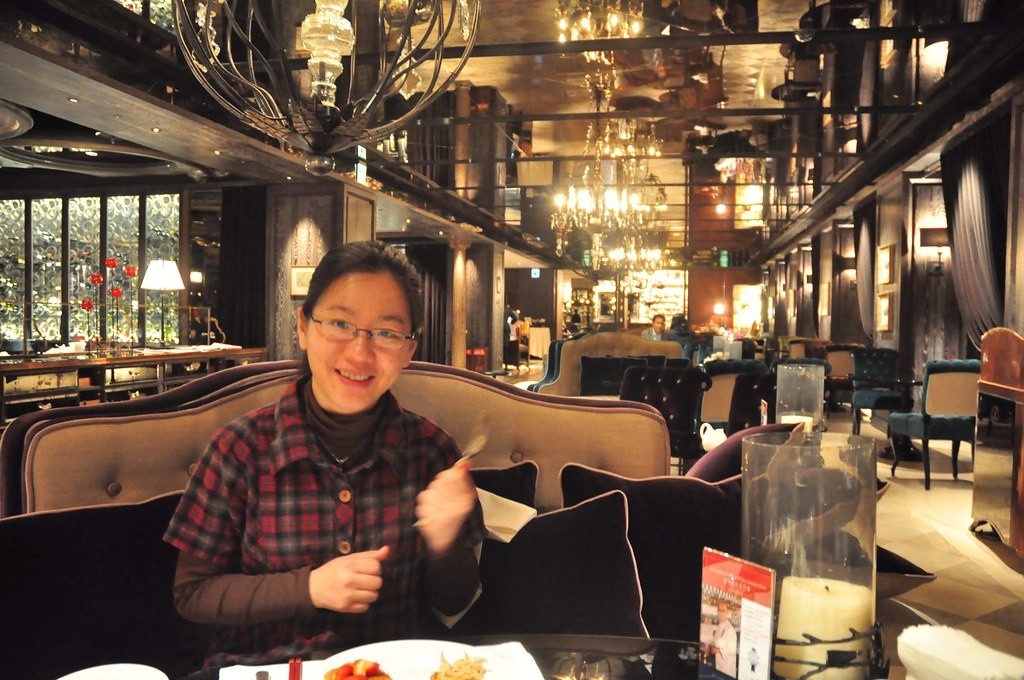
[{"left": 218, "top": 638, "right": 544, "bottom": 680}]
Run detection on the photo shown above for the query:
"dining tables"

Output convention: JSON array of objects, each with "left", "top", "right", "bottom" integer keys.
[{"left": 171, "top": 633, "right": 867, "bottom": 680}]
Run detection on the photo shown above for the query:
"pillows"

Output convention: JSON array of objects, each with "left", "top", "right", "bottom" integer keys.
[
  {"left": 440, "top": 489, "right": 655, "bottom": 663},
  {"left": 564, "top": 465, "right": 762, "bottom": 634}
]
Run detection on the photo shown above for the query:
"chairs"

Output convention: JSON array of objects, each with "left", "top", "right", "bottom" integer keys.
[
  {"left": 886, "top": 358, "right": 978, "bottom": 490},
  {"left": 844, "top": 346, "right": 919, "bottom": 467}
]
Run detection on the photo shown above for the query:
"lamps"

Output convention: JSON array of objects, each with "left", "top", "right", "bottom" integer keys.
[
  {"left": 545, "top": 1, "right": 674, "bottom": 277},
  {"left": 173, "top": 1, "right": 481, "bottom": 178}
]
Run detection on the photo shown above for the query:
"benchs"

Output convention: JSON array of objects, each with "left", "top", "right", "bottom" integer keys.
[{"left": 0, "top": 323, "right": 866, "bottom": 680}]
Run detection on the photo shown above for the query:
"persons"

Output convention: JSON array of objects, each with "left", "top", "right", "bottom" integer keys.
[
  {"left": 641, "top": 315, "right": 668, "bottom": 341},
  {"left": 712, "top": 603, "right": 737, "bottom": 678},
  {"left": 164, "top": 241, "right": 488, "bottom": 679},
  {"left": 664, "top": 314, "right": 699, "bottom": 361},
  {"left": 504, "top": 306, "right": 517, "bottom": 362},
  {"left": 571, "top": 309, "right": 580, "bottom": 323},
  {"left": 749, "top": 648, "right": 759, "bottom": 671}
]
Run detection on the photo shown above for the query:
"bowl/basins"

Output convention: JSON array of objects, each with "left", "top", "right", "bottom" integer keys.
[{"left": 56, "top": 662, "right": 170, "bottom": 680}]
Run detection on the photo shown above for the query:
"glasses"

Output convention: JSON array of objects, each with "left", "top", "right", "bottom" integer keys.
[{"left": 305, "top": 311, "right": 417, "bottom": 349}]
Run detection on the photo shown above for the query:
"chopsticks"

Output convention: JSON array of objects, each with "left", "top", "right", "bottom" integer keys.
[{"left": 288, "top": 657, "right": 301, "bottom": 680}]
